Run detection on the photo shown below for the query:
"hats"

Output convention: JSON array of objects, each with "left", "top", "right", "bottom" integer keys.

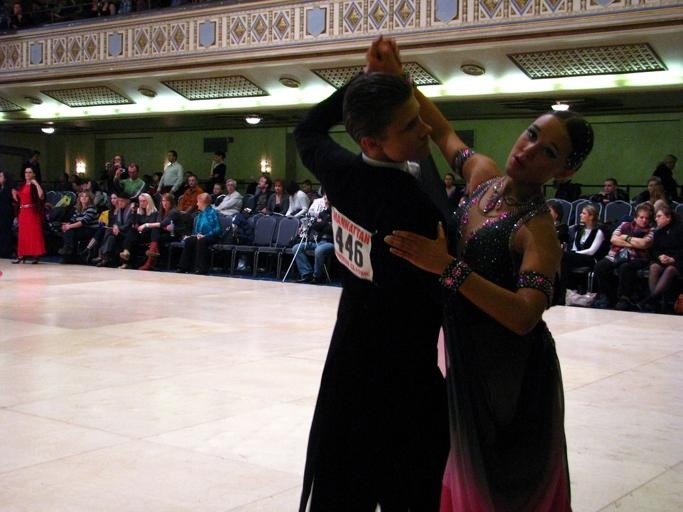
[{"left": 118, "top": 191, "right": 130, "bottom": 199}]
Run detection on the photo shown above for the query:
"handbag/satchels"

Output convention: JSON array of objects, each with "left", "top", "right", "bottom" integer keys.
[
  {"left": 565, "top": 288, "right": 598, "bottom": 308},
  {"left": 612, "top": 248, "right": 628, "bottom": 266},
  {"left": 54, "top": 195, "right": 71, "bottom": 208}
]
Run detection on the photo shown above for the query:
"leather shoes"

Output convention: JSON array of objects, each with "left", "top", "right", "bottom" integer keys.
[
  {"left": 298, "top": 275, "right": 312, "bottom": 283},
  {"left": 311, "top": 278, "right": 321, "bottom": 284}
]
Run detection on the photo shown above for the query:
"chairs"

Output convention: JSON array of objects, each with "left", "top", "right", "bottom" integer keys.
[
  {"left": 169, "top": 193, "right": 301, "bottom": 281},
  {"left": 546, "top": 193, "right": 683, "bottom": 303}
]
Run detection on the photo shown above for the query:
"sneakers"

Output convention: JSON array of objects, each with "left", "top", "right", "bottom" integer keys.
[{"left": 58, "top": 241, "right": 160, "bottom": 270}]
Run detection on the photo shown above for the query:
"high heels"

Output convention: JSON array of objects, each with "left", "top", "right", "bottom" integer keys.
[
  {"left": 12, "top": 256, "right": 25, "bottom": 264},
  {"left": 32, "top": 257, "right": 40, "bottom": 264}
]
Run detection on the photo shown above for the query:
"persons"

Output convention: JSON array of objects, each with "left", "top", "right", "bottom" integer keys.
[
  {"left": 542, "top": 151, "right": 682, "bottom": 310},
  {"left": 0, "top": 0, "right": 153, "bottom": 30},
  {"left": 382, "top": 38, "right": 594, "bottom": 510},
  {"left": 292, "top": 34, "right": 451, "bottom": 512},
  {"left": 0, "top": 150, "right": 340, "bottom": 285},
  {"left": 443, "top": 173, "right": 463, "bottom": 212}
]
